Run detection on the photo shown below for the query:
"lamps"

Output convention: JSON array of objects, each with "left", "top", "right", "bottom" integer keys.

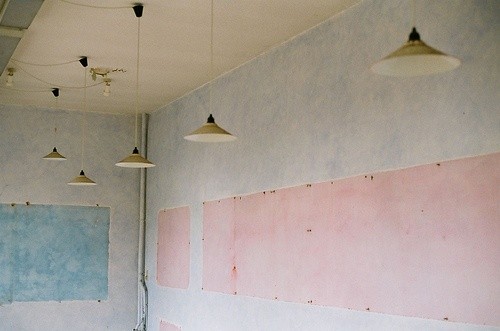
[
  {"left": 104, "top": 78, "right": 112, "bottom": 96},
  {"left": 43, "top": 88, "right": 66, "bottom": 160},
  {"left": 116, "top": 5, "right": 155, "bottom": 168},
  {"left": 67, "top": 57, "right": 97, "bottom": 186},
  {"left": 183, "top": 0, "right": 237, "bottom": 142},
  {"left": 371, "top": 0, "right": 462, "bottom": 76},
  {"left": 6, "top": 67, "right": 16, "bottom": 87}
]
